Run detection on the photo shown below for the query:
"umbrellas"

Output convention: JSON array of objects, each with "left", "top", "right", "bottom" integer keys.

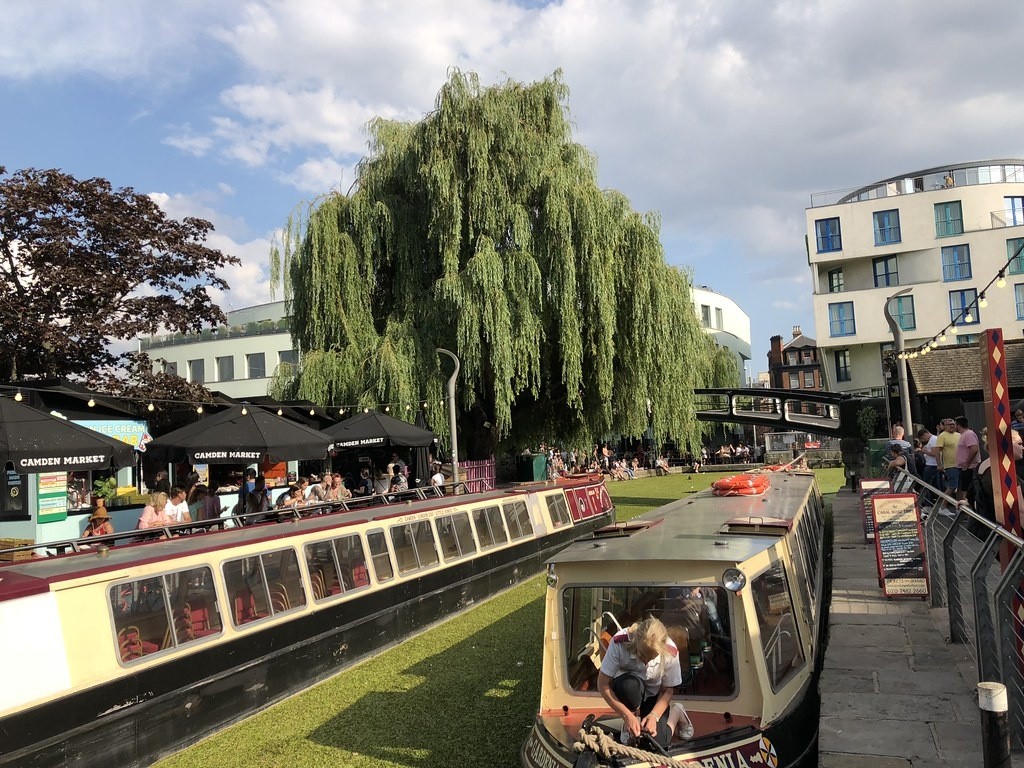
[
  {"left": 0, "top": 396, "right": 136, "bottom": 475},
  {"left": 321, "top": 410, "right": 436, "bottom": 493},
  {"left": 145, "top": 400, "right": 332, "bottom": 524}
]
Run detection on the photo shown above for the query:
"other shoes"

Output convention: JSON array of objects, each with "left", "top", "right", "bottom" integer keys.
[
  {"left": 617, "top": 476, "right": 626, "bottom": 481},
  {"left": 629, "top": 476, "right": 638, "bottom": 480},
  {"left": 693, "top": 467, "right": 696, "bottom": 469},
  {"left": 695, "top": 469, "right": 698, "bottom": 473},
  {"left": 620, "top": 722, "right": 629, "bottom": 744},
  {"left": 920, "top": 513, "right": 927, "bottom": 524},
  {"left": 667, "top": 471, "right": 673, "bottom": 475},
  {"left": 920, "top": 506, "right": 932, "bottom": 513},
  {"left": 938, "top": 508, "right": 955, "bottom": 516},
  {"left": 955, "top": 510, "right": 968, "bottom": 522},
  {"left": 670, "top": 702, "right": 694, "bottom": 740}
]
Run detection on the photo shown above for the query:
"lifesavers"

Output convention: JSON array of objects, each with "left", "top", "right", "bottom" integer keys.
[
  {"left": 760, "top": 463, "right": 791, "bottom": 472},
  {"left": 711, "top": 473, "right": 770, "bottom": 495}
]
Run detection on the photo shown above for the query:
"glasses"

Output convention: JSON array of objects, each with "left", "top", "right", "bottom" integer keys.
[{"left": 946, "top": 423, "right": 955, "bottom": 426}]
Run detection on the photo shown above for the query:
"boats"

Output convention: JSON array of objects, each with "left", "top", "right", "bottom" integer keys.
[
  {"left": 519, "top": 465, "right": 831, "bottom": 768},
  {"left": 1, "top": 462, "right": 620, "bottom": 755}
]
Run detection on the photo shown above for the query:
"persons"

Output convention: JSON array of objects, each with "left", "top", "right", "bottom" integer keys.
[
  {"left": 885, "top": 416, "right": 1024, "bottom": 561},
  {"left": 678, "top": 587, "right": 722, "bottom": 635},
  {"left": 524, "top": 440, "right": 750, "bottom": 480},
  {"left": 80, "top": 507, "right": 114, "bottom": 546},
  {"left": 130, "top": 471, "right": 228, "bottom": 544},
  {"left": 791, "top": 443, "right": 799, "bottom": 460},
  {"left": 431, "top": 465, "right": 444, "bottom": 496},
  {"left": 943, "top": 176, "right": 953, "bottom": 185},
  {"left": 354, "top": 453, "right": 410, "bottom": 497},
  {"left": 598, "top": 618, "right": 694, "bottom": 748},
  {"left": 242, "top": 467, "right": 352, "bottom": 523}
]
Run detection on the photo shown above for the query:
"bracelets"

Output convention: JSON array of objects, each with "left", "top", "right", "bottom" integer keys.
[{"left": 651, "top": 712, "right": 659, "bottom": 722}]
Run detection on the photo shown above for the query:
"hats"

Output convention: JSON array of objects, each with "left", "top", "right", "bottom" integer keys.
[{"left": 87, "top": 506, "right": 112, "bottom": 523}]
